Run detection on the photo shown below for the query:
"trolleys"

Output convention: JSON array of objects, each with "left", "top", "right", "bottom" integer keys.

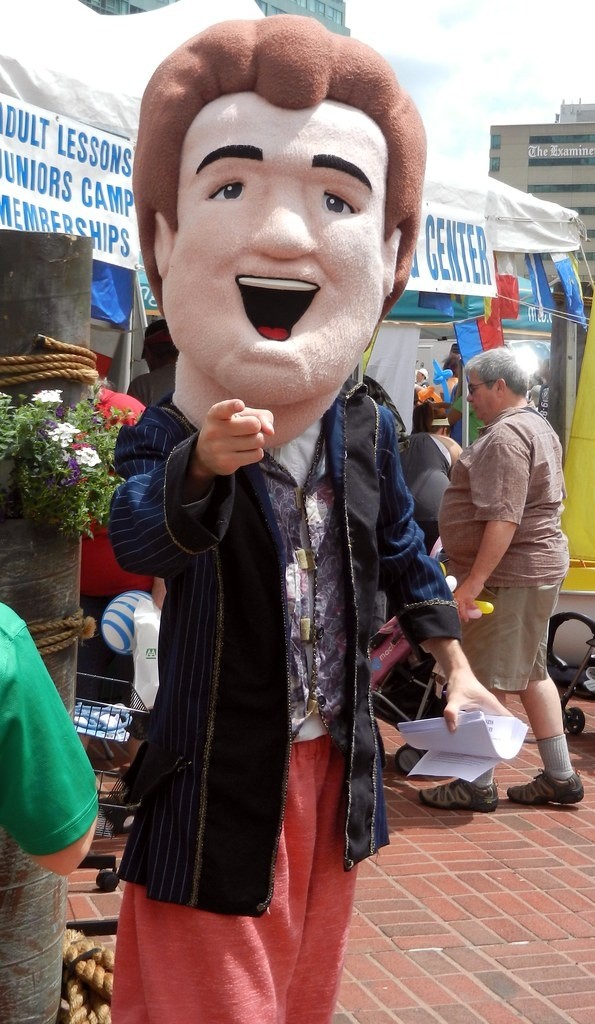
[{"left": 70, "top": 668, "right": 150, "bottom": 893}]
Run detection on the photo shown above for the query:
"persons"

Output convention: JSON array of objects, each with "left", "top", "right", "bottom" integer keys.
[
  {"left": 76, "top": 318, "right": 585, "bottom": 832},
  {"left": 0, "top": 602, "right": 98, "bottom": 877},
  {"left": 106, "top": 13, "right": 519, "bottom": 1024}
]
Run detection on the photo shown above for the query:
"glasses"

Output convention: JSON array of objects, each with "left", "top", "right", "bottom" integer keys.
[{"left": 468, "top": 379, "right": 507, "bottom": 395}]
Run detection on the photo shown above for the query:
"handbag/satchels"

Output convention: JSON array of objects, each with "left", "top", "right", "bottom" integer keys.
[{"left": 131, "top": 598, "right": 162, "bottom": 710}]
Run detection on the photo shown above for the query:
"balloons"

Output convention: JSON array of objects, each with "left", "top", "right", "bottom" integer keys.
[
  {"left": 417, "top": 385, "right": 445, "bottom": 412},
  {"left": 432, "top": 357, "right": 453, "bottom": 412}
]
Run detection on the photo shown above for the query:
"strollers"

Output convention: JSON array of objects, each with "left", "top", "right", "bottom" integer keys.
[
  {"left": 369, "top": 536, "right": 447, "bottom": 775},
  {"left": 548, "top": 610, "right": 595, "bottom": 735}
]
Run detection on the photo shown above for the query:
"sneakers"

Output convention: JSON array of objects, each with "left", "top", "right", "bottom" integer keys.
[
  {"left": 507, "top": 768, "right": 584, "bottom": 805},
  {"left": 419, "top": 778, "right": 498, "bottom": 812}
]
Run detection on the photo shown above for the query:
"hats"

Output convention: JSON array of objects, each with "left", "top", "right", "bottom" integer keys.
[
  {"left": 141, "top": 331, "right": 172, "bottom": 359},
  {"left": 418, "top": 368, "right": 428, "bottom": 379}
]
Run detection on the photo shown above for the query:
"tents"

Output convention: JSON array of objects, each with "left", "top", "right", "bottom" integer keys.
[{"left": 0, "top": 50, "right": 587, "bottom": 462}]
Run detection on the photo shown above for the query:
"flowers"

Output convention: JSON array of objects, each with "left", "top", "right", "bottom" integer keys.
[{"left": 0, "top": 380, "right": 142, "bottom": 542}]
